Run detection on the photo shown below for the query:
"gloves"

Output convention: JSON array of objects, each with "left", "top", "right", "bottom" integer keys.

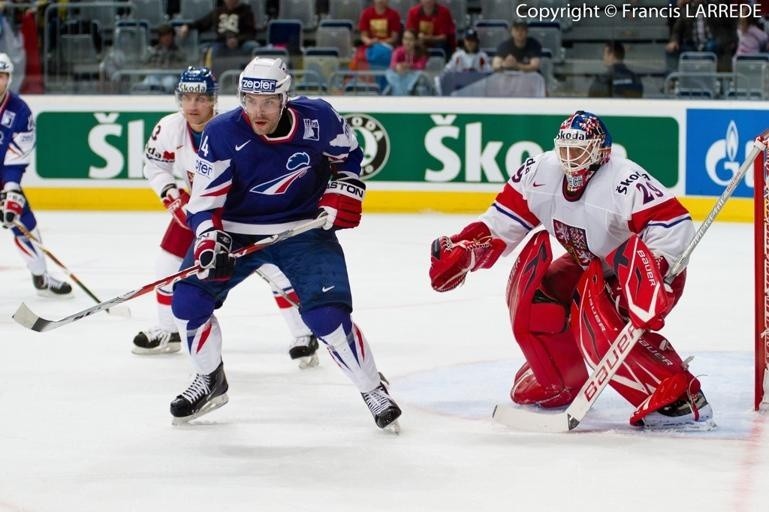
[
  {"left": 315, "top": 173, "right": 366, "bottom": 232},
  {"left": 193, "top": 229, "right": 236, "bottom": 282},
  {"left": 161, "top": 188, "right": 194, "bottom": 231},
  {"left": 0, "top": 190, "right": 27, "bottom": 230}
]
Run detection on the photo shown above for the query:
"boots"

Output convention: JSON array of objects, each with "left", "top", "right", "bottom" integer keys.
[
  {"left": 171, "top": 361, "right": 228, "bottom": 417},
  {"left": 33, "top": 272, "right": 72, "bottom": 294},
  {"left": 360, "top": 382, "right": 402, "bottom": 429}
]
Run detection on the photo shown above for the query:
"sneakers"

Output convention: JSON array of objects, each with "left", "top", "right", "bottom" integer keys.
[
  {"left": 289, "top": 336, "right": 319, "bottom": 360},
  {"left": 637, "top": 388, "right": 712, "bottom": 426},
  {"left": 133, "top": 329, "right": 182, "bottom": 348}
]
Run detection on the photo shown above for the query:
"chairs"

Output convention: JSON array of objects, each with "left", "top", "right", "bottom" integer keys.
[{"left": 38, "top": 1, "right": 769, "bottom": 98}]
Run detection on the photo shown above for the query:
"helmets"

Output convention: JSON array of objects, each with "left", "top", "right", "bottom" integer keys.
[
  {"left": 236, "top": 57, "right": 293, "bottom": 116},
  {"left": 553, "top": 111, "right": 612, "bottom": 192},
  {"left": 175, "top": 67, "right": 217, "bottom": 110},
  {"left": 0, "top": 53, "right": 14, "bottom": 88}
]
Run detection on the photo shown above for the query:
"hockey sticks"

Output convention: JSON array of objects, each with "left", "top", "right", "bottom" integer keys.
[
  {"left": 11, "top": 216, "right": 327, "bottom": 332},
  {"left": 492, "top": 140, "right": 766, "bottom": 432},
  {"left": 14, "top": 218, "right": 131, "bottom": 316}
]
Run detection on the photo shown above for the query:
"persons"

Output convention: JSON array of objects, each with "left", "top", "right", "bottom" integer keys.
[
  {"left": 131, "top": 68, "right": 319, "bottom": 368},
  {"left": 179, "top": 0, "right": 261, "bottom": 63},
  {"left": 384, "top": 29, "right": 427, "bottom": 95},
  {"left": 429, "top": 110, "right": 716, "bottom": 430},
  {"left": 405, "top": 0, "right": 456, "bottom": 60},
  {"left": 0, "top": 51, "right": 72, "bottom": 294},
  {"left": 491, "top": 17, "right": 549, "bottom": 96},
  {"left": 436, "top": 28, "right": 493, "bottom": 95},
  {"left": 170, "top": 57, "right": 402, "bottom": 432},
  {"left": 359, "top": 0, "right": 401, "bottom": 83},
  {"left": 142, "top": 24, "right": 183, "bottom": 92},
  {"left": 589, "top": 40, "right": 644, "bottom": 97},
  {"left": 659, "top": 1, "right": 769, "bottom": 92}
]
